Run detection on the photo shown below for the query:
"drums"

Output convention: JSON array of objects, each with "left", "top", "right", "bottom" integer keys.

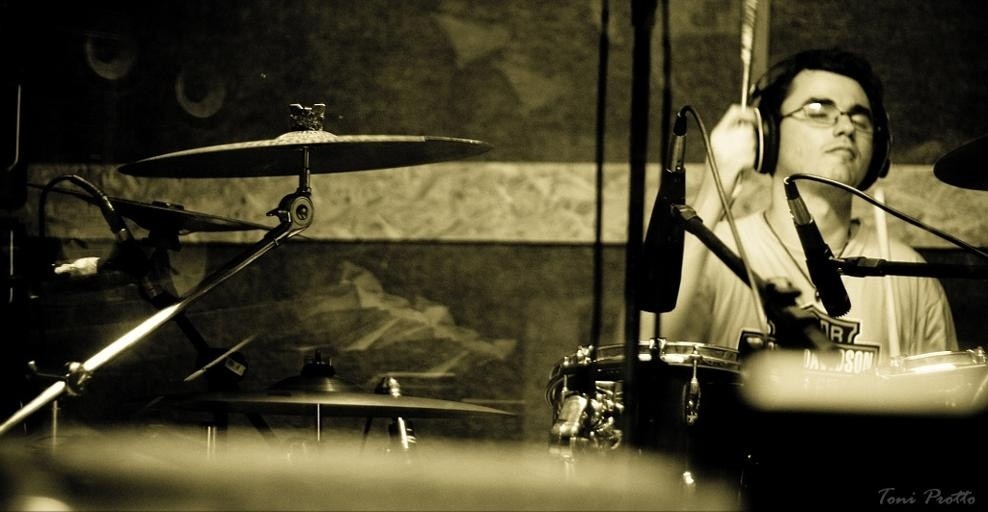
[
  {"left": 543, "top": 338, "right": 745, "bottom": 486},
  {"left": 30, "top": 296, "right": 153, "bottom": 371}
]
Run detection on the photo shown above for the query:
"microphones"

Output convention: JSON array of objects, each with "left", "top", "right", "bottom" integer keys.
[
  {"left": 68, "top": 172, "right": 173, "bottom": 308},
  {"left": 779, "top": 174, "right": 854, "bottom": 320},
  {"left": 630, "top": 113, "right": 690, "bottom": 315}
]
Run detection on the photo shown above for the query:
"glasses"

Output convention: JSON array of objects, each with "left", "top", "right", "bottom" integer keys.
[{"left": 780, "top": 100, "right": 883, "bottom": 137}]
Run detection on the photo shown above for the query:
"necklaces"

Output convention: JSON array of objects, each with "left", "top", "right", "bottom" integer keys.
[{"left": 762, "top": 210, "right": 852, "bottom": 302}]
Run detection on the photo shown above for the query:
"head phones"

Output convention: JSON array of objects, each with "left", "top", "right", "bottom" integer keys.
[{"left": 745, "top": 44, "right": 896, "bottom": 192}]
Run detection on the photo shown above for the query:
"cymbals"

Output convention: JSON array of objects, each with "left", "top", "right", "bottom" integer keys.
[
  {"left": 934, "top": 132, "right": 987, "bottom": 192},
  {"left": 171, "top": 374, "right": 520, "bottom": 419},
  {"left": 23, "top": 181, "right": 273, "bottom": 233},
  {"left": 118, "top": 130, "right": 493, "bottom": 178}
]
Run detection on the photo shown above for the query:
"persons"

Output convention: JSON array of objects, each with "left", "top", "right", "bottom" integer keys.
[{"left": 614, "top": 47, "right": 959, "bottom": 378}]
